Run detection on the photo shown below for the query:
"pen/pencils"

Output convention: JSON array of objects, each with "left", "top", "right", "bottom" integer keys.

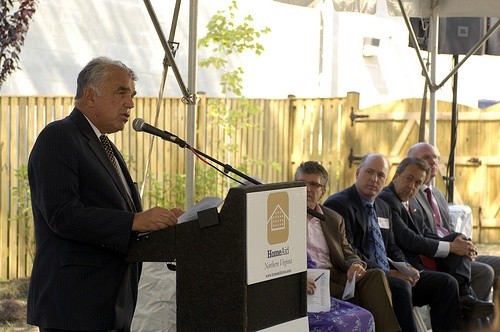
[{"left": 314, "top": 272, "right": 324, "bottom": 282}]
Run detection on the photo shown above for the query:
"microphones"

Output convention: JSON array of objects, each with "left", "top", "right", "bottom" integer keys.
[{"left": 132, "top": 118, "right": 182, "bottom": 143}]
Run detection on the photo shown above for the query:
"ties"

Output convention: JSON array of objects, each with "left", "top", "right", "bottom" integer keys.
[
  {"left": 424, "top": 187, "right": 449, "bottom": 236},
  {"left": 365, "top": 201, "right": 391, "bottom": 274},
  {"left": 98, "top": 134, "right": 119, "bottom": 172}
]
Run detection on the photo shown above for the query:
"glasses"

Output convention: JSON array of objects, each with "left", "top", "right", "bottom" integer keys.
[{"left": 295, "top": 180, "right": 326, "bottom": 191}]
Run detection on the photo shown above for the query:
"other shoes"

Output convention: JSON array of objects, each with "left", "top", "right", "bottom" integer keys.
[{"left": 460, "top": 293, "right": 494, "bottom": 319}]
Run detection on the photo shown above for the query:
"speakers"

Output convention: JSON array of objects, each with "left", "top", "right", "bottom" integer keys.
[{"left": 408, "top": 17, "right": 500, "bottom": 57}]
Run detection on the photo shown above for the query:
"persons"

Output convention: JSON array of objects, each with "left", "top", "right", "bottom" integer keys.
[
  {"left": 294, "top": 161, "right": 402, "bottom": 332},
  {"left": 235, "top": 178, "right": 376, "bottom": 332},
  {"left": 323, "top": 142, "right": 500, "bottom": 332},
  {"left": 27, "top": 57, "right": 183, "bottom": 332}
]
